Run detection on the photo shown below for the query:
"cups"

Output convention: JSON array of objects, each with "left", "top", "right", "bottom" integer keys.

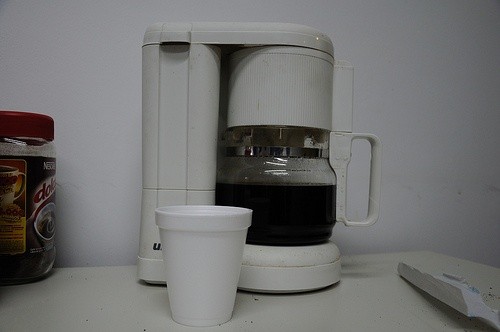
[{"left": 156, "top": 205, "right": 253, "bottom": 326}]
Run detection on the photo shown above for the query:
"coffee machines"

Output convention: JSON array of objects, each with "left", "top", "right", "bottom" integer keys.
[{"left": 138, "top": 22, "right": 383, "bottom": 294}]
